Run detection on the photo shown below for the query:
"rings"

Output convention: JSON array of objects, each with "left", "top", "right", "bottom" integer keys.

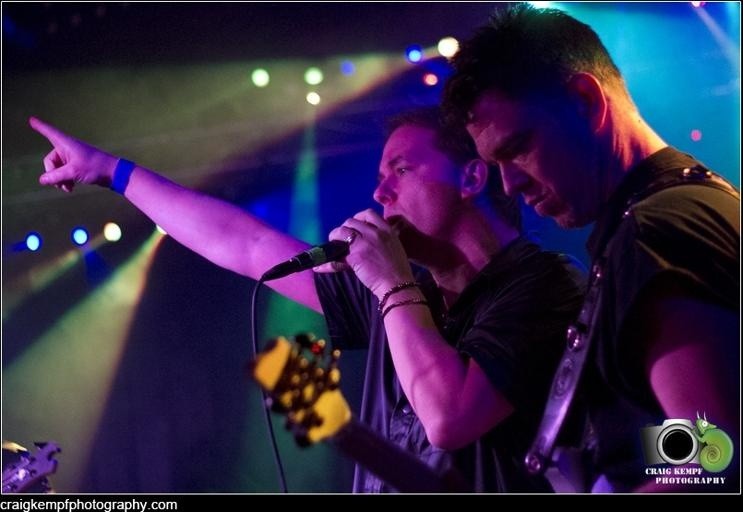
[{"left": 342, "top": 229, "right": 359, "bottom": 247}]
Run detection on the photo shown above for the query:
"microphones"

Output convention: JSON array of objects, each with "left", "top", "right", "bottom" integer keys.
[{"left": 258, "top": 221, "right": 401, "bottom": 279}]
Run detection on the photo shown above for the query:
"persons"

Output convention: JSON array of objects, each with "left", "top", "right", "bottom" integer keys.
[
  {"left": 21, "top": 87, "right": 592, "bottom": 497},
  {"left": 434, "top": 2, "right": 737, "bottom": 491}
]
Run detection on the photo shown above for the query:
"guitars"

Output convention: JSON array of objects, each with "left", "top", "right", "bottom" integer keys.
[{"left": 248, "top": 330, "right": 584, "bottom": 494}]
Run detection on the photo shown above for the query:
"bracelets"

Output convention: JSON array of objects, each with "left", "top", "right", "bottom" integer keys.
[
  {"left": 374, "top": 298, "right": 431, "bottom": 318},
  {"left": 367, "top": 280, "right": 425, "bottom": 307},
  {"left": 104, "top": 155, "right": 137, "bottom": 197}
]
